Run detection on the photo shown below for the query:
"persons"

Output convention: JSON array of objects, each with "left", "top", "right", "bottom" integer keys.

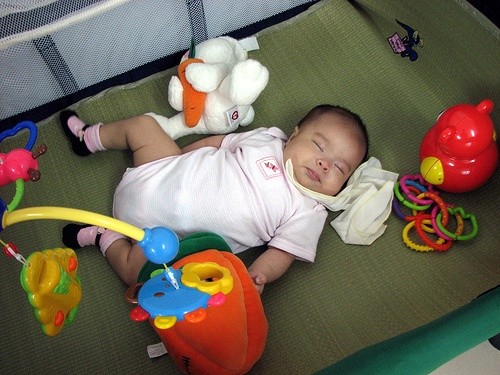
[{"left": 58, "top": 103, "right": 370, "bottom": 298}]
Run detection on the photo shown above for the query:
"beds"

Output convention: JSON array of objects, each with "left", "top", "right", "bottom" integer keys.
[{"left": 0, "top": 1, "right": 500, "bottom": 375}]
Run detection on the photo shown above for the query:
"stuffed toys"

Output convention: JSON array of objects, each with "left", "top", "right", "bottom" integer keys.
[
  {"left": 142, "top": 35, "right": 269, "bottom": 142},
  {"left": 134, "top": 231, "right": 269, "bottom": 375}
]
